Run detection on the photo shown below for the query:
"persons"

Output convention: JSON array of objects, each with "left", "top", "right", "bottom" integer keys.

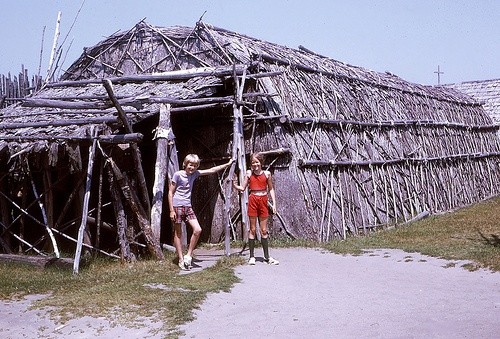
[
  {"left": 232, "top": 153, "right": 280, "bottom": 265},
  {"left": 168, "top": 153, "right": 235, "bottom": 270}
]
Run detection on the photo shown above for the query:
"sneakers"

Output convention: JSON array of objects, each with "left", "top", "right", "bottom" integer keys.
[
  {"left": 263, "top": 257, "right": 279, "bottom": 265},
  {"left": 179, "top": 255, "right": 192, "bottom": 271},
  {"left": 248, "top": 258, "right": 255, "bottom": 264}
]
